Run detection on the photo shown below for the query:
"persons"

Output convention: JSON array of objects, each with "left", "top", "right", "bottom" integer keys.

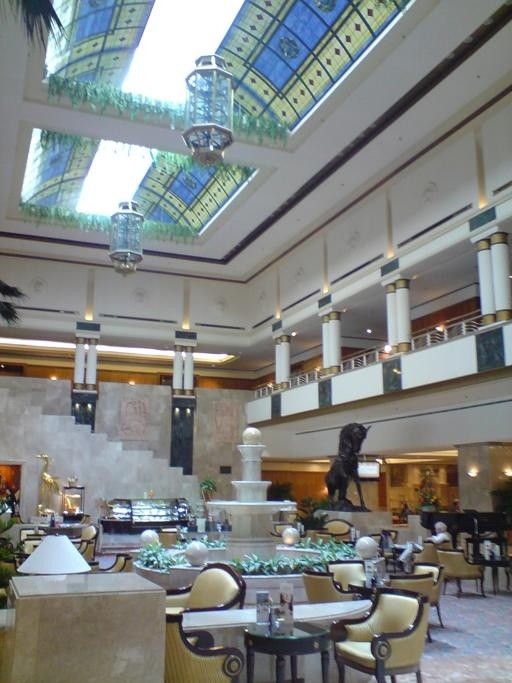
[
  {"left": 450, "top": 498, "right": 460, "bottom": 512},
  {"left": 400, "top": 502, "right": 411, "bottom": 522}
]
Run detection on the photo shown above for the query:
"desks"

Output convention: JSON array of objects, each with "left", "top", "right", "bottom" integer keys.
[{"left": 242, "top": 622, "right": 331, "bottom": 682}]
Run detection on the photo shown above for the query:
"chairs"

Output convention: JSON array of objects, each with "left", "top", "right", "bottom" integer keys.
[
  {"left": 165, "top": 612, "right": 245, "bottom": 682},
  {"left": 273, "top": 512, "right": 512, "bottom": 604},
  {"left": 165, "top": 563, "right": 246, "bottom": 613},
  {"left": 413, "top": 562, "right": 445, "bottom": 629},
  {"left": 157, "top": 525, "right": 180, "bottom": 546},
  {"left": 389, "top": 573, "right": 434, "bottom": 643},
  {"left": 1, "top": 510, "right": 134, "bottom": 574},
  {"left": 332, "top": 588, "right": 430, "bottom": 682}
]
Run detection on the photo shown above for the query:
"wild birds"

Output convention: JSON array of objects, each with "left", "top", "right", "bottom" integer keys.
[{"left": 35, "top": 453, "right": 62, "bottom": 510}]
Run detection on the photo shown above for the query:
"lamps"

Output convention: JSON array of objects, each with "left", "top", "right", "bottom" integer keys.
[
  {"left": 180, "top": 53, "right": 237, "bottom": 168},
  {"left": 108, "top": 201, "right": 145, "bottom": 279}
]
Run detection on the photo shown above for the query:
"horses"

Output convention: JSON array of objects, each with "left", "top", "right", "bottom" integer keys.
[{"left": 324, "top": 421, "right": 373, "bottom": 512}]
